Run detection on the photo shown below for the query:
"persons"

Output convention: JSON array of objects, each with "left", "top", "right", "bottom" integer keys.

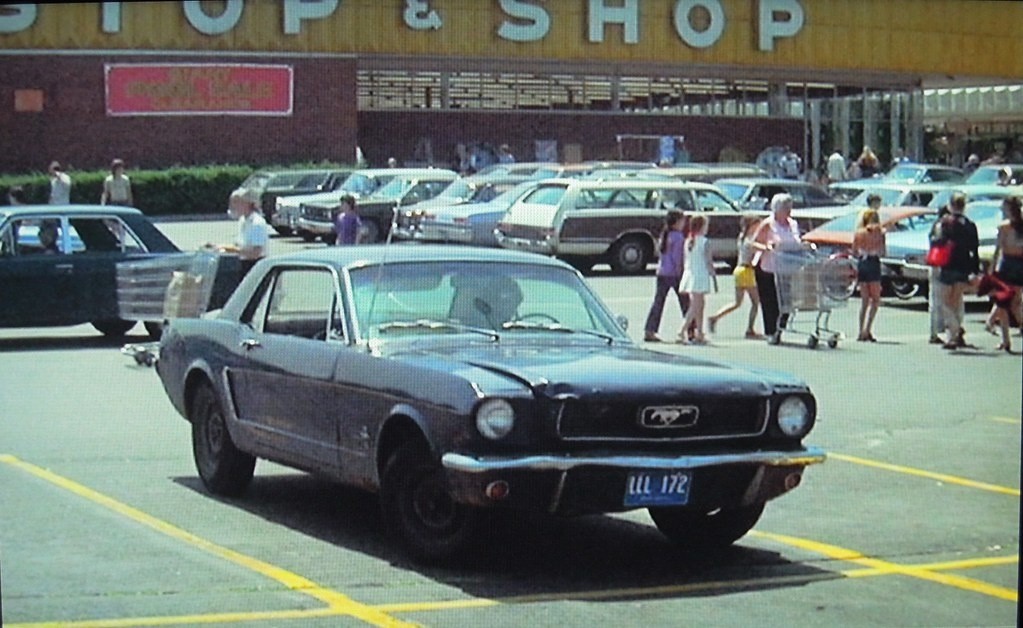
[
  {"left": 674, "top": 214, "right": 718, "bottom": 346},
  {"left": 757, "top": 140, "right": 1023, "bottom": 195},
  {"left": 46, "top": 160, "right": 73, "bottom": 204},
  {"left": 989, "top": 194, "right": 1023, "bottom": 352},
  {"left": 929, "top": 190, "right": 980, "bottom": 351},
  {"left": 927, "top": 205, "right": 951, "bottom": 346},
  {"left": 458, "top": 142, "right": 516, "bottom": 176},
  {"left": 748, "top": 194, "right": 815, "bottom": 346},
  {"left": 851, "top": 210, "right": 887, "bottom": 342},
  {"left": 204, "top": 187, "right": 270, "bottom": 260},
  {"left": 644, "top": 209, "right": 698, "bottom": 342},
  {"left": 982, "top": 300, "right": 1003, "bottom": 336},
  {"left": 706, "top": 214, "right": 769, "bottom": 340},
  {"left": 8, "top": 182, "right": 33, "bottom": 206},
  {"left": 36, "top": 222, "right": 59, "bottom": 254},
  {"left": 99, "top": 158, "right": 133, "bottom": 244},
  {"left": 333, "top": 194, "right": 362, "bottom": 248},
  {"left": 853, "top": 192, "right": 883, "bottom": 231}
]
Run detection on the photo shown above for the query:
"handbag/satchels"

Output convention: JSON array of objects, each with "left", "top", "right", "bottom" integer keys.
[
  {"left": 987, "top": 269, "right": 1014, "bottom": 308},
  {"left": 925, "top": 239, "right": 957, "bottom": 268}
]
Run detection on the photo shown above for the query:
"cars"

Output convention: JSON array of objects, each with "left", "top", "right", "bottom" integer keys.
[
  {"left": 156, "top": 244, "right": 824, "bottom": 555},
  {"left": 224, "top": 156, "right": 1022, "bottom": 299},
  {"left": 0, "top": 205, "right": 193, "bottom": 340}
]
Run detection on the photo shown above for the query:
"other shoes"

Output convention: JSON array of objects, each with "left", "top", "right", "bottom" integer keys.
[{"left": 644, "top": 312, "right": 1022, "bottom": 355}]
